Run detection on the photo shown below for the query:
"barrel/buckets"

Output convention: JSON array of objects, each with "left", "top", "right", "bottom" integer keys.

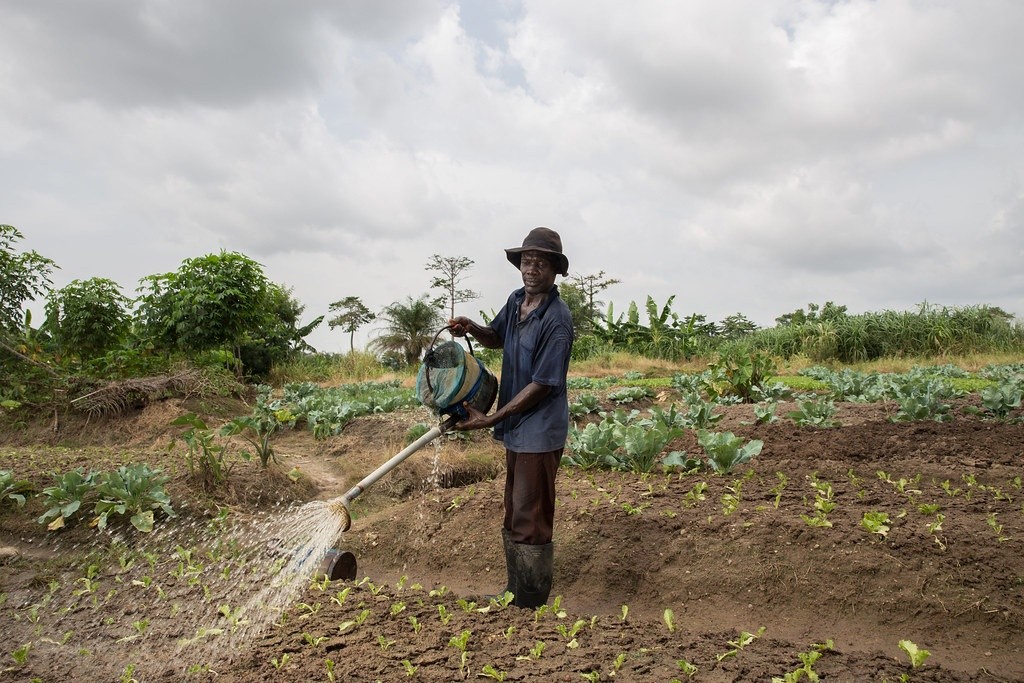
[{"left": 267, "top": 539, "right": 357, "bottom": 581}]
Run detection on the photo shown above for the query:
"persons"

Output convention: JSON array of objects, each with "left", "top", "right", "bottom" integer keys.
[{"left": 448, "top": 228, "right": 574, "bottom": 607}]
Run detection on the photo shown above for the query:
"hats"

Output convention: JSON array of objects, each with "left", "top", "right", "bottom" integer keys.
[{"left": 505, "top": 227, "right": 569, "bottom": 275}]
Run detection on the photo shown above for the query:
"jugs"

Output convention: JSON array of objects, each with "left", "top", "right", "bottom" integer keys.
[{"left": 248, "top": 325, "right": 498, "bottom": 532}]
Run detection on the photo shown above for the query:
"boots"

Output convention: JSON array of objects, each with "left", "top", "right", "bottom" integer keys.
[
  {"left": 514, "top": 542, "right": 554, "bottom": 610},
  {"left": 482, "top": 528, "right": 514, "bottom": 606}
]
